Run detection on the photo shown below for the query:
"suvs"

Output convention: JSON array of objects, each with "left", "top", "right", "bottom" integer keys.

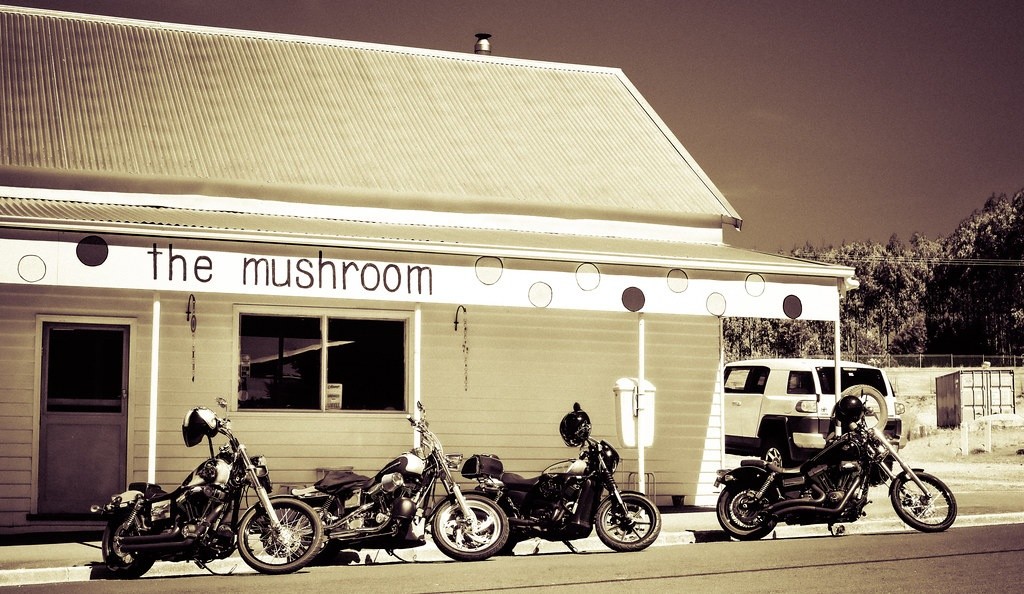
[{"left": 722, "top": 358, "right": 902, "bottom": 465}]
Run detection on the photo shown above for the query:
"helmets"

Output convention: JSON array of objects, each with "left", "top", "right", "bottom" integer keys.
[
  {"left": 183, "top": 407, "right": 219, "bottom": 447},
  {"left": 836, "top": 395, "right": 862, "bottom": 423},
  {"left": 560, "top": 409, "right": 591, "bottom": 447}
]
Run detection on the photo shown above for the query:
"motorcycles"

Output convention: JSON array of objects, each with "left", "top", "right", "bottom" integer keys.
[
  {"left": 711, "top": 388, "right": 959, "bottom": 540},
  {"left": 90, "top": 396, "right": 324, "bottom": 576},
  {"left": 273, "top": 398, "right": 513, "bottom": 562},
  {"left": 458, "top": 402, "right": 662, "bottom": 554}
]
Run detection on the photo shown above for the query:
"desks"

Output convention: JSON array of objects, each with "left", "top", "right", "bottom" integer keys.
[{"left": 316, "top": 467, "right": 353, "bottom": 483}]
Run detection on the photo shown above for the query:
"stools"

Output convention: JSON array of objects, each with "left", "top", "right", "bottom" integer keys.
[
  {"left": 277, "top": 484, "right": 306, "bottom": 495},
  {"left": 628, "top": 472, "right": 657, "bottom": 505}
]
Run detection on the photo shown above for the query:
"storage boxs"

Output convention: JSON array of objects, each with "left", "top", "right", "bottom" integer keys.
[{"left": 935, "top": 370, "right": 1015, "bottom": 429}]
